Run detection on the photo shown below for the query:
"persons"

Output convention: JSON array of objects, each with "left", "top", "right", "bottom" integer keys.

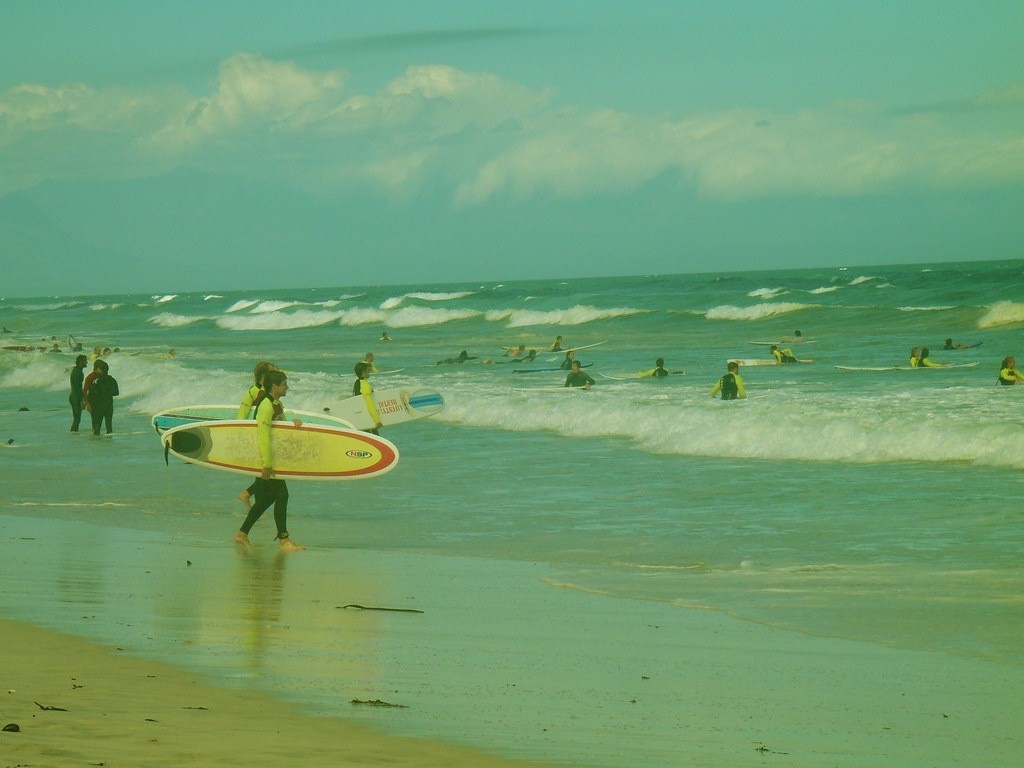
[
  {"left": 710, "top": 362, "right": 747, "bottom": 400},
  {"left": 769, "top": 346, "right": 799, "bottom": 363},
  {"left": 549, "top": 335, "right": 562, "bottom": 352},
  {"left": 918, "top": 347, "right": 944, "bottom": 367},
  {"left": 352, "top": 362, "right": 384, "bottom": 436},
  {"left": 69, "top": 355, "right": 119, "bottom": 435},
  {"left": 161, "top": 349, "right": 175, "bottom": 359},
  {"left": 782, "top": 330, "right": 805, "bottom": 343},
  {"left": 1, "top": 328, "right": 24, "bottom": 333},
  {"left": 235, "top": 361, "right": 306, "bottom": 550},
  {"left": 90, "top": 346, "right": 120, "bottom": 360},
  {"left": 944, "top": 339, "right": 959, "bottom": 349},
  {"left": 564, "top": 361, "right": 595, "bottom": 387},
  {"left": 561, "top": 351, "right": 574, "bottom": 370},
  {"left": 909, "top": 347, "right": 920, "bottom": 367},
  {"left": 363, "top": 352, "right": 380, "bottom": 373},
  {"left": 638, "top": 357, "right": 669, "bottom": 378},
  {"left": 500, "top": 345, "right": 526, "bottom": 358},
  {"left": 482, "top": 349, "right": 536, "bottom": 366},
  {"left": 39, "top": 334, "right": 85, "bottom": 352},
  {"left": 437, "top": 351, "right": 478, "bottom": 365},
  {"left": 999, "top": 356, "right": 1024, "bottom": 385},
  {"left": 379, "top": 332, "right": 392, "bottom": 341}
]
